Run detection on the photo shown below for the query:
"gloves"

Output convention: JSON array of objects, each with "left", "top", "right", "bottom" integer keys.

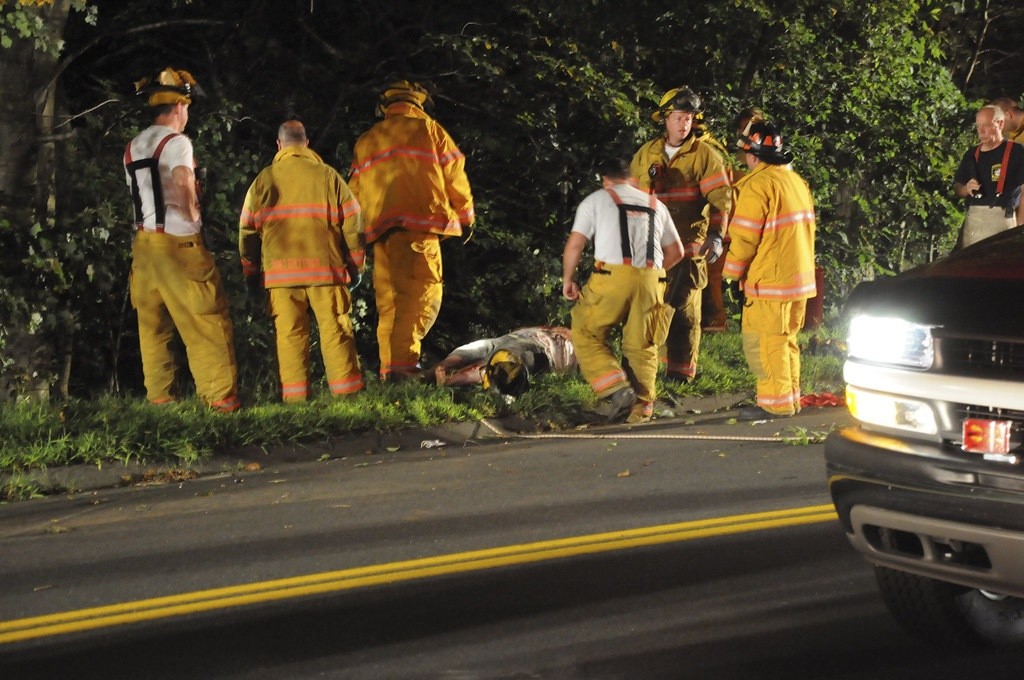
[
  {"left": 347, "top": 270, "right": 364, "bottom": 293},
  {"left": 461, "top": 223, "right": 476, "bottom": 244},
  {"left": 699, "top": 230, "right": 724, "bottom": 264},
  {"left": 721, "top": 278, "right": 744, "bottom": 325},
  {"left": 244, "top": 272, "right": 261, "bottom": 295}
]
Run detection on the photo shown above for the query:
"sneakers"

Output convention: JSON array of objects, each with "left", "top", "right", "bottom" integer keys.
[
  {"left": 738, "top": 398, "right": 797, "bottom": 422},
  {"left": 606, "top": 386, "right": 653, "bottom": 424}
]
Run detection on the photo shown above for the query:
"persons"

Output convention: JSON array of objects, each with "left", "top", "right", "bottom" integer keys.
[
  {"left": 423, "top": 326, "right": 576, "bottom": 380},
  {"left": 952, "top": 97, "right": 1024, "bottom": 247},
  {"left": 123, "top": 70, "right": 241, "bottom": 412},
  {"left": 563, "top": 158, "right": 686, "bottom": 427},
  {"left": 632, "top": 88, "right": 816, "bottom": 419},
  {"left": 349, "top": 80, "right": 475, "bottom": 379},
  {"left": 239, "top": 122, "right": 368, "bottom": 405}
]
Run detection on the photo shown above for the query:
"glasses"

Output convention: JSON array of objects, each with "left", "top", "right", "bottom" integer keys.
[{"left": 672, "top": 91, "right": 702, "bottom": 111}]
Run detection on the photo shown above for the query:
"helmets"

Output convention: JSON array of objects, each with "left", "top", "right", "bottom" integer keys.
[
  {"left": 735, "top": 108, "right": 766, "bottom": 122},
  {"left": 652, "top": 89, "right": 703, "bottom": 122},
  {"left": 134, "top": 67, "right": 197, "bottom": 106},
  {"left": 384, "top": 80, "right": 427, "bottom": 110},
  {"left": 736, "top": 123, "right": 794, "bottom": 165},
  {"left": 479, "top": 349, "right": 529, "bottom": 396}
]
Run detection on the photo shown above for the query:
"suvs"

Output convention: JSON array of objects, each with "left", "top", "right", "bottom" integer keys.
[{"left": 822, "top": 224, "right": 1024, "bottom": 641}]
